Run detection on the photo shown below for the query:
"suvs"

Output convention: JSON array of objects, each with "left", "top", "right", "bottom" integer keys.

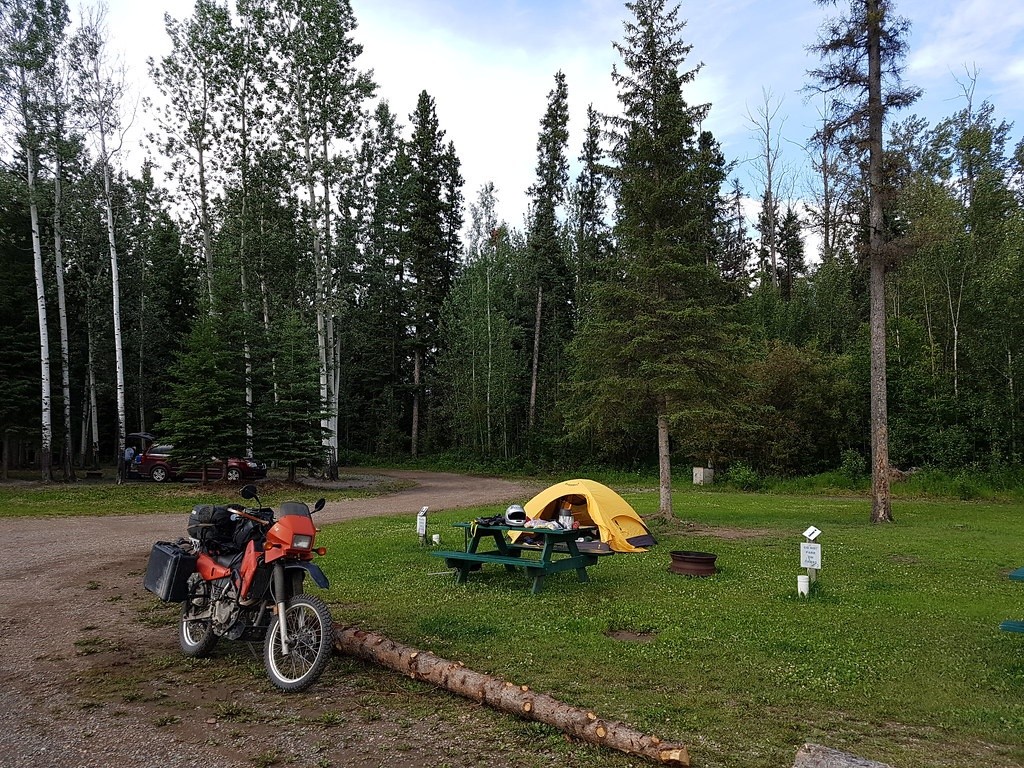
[{"left": 127, "top": 432, "right": 268, "bottom": 481}]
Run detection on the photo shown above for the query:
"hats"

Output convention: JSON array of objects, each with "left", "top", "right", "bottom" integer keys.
[{"left": 133, "top": 446, "right": 136, "bottom": 450}]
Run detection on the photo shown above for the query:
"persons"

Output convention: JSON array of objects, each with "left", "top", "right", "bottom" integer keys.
[{"left": 125, "top": 447, "right": 136, "bottom": 478}]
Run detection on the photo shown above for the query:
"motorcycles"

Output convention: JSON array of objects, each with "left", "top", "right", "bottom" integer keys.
[{"left": 144, "top": 484, "right": 335, "bottom": 694}]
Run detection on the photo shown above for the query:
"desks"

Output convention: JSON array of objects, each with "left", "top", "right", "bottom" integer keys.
[{"left": 452, "top": 524, "right": 597, "bottom": 596}]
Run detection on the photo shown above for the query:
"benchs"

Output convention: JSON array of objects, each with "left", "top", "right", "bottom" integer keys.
[
  {"left": 432, "top": 551, "right": 553, "bottom": 568},
  {"left": 505, "top": 544, "right": 615, "bottom": 556}
]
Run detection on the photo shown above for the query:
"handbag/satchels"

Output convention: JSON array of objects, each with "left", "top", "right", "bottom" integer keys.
[
  {"left": 470, "top": 514, "right": 505, "bottom": 537},
  {"left": 187, "top": 504, "right": 274, "bottom": 558}
]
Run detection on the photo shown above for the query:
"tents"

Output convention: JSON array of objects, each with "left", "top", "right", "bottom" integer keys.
[{"left": 503, "top": 479, "right": 657, "bottom": 552}]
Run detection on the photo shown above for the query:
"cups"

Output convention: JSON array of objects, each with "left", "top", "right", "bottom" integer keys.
[
  {"left": 567, "top": 516, "right": 574, "bottom": 529},
  {"left": 559, "top": 509, "right": 571, "bottom": 529}
]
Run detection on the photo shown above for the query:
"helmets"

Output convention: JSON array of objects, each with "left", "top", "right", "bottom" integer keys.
[{"left": 504, "top": 505, "right": 526, "bottom": 526}]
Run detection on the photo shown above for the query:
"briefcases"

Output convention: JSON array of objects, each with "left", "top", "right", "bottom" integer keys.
[{"left": 144, "top": 541, "right": 197, "bottom": 603}]
